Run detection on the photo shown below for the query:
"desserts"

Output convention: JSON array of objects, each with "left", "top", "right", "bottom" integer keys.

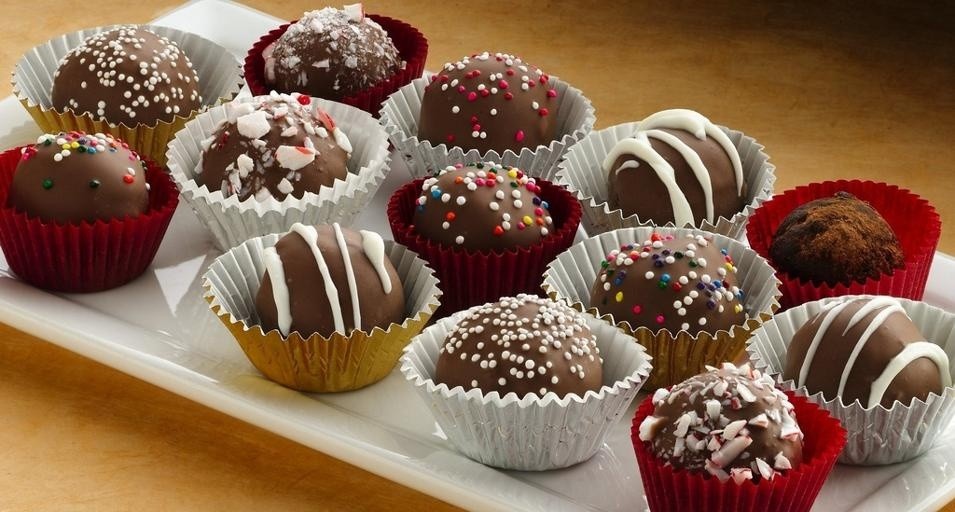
[{"left": 0, "top": 1, "right": 955, "bottom": 512}]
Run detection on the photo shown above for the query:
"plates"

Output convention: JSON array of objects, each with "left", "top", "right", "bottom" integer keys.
[{"left": 1, "top": 0, "right": 949, "bottom": 511}]
